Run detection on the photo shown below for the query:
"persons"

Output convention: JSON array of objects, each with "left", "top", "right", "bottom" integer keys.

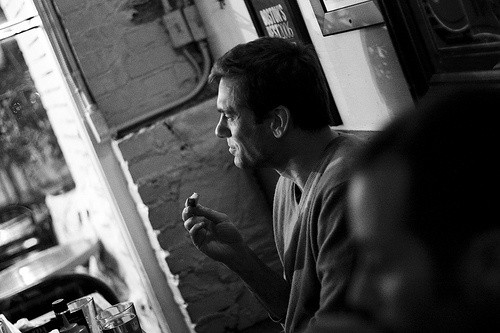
[
  {"left": 346, "top": 86, "right": 500, "bottom": 333},
  {"left": 183, "top": 39, "right": 379, "bottom": 333}
]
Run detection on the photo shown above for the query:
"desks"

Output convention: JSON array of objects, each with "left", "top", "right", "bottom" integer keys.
[{"left": 0, "top": 238, "right": 100, "bottom": 309}]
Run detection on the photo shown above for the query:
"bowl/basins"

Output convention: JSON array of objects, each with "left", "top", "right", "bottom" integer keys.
[{"left": 19, "top": 296, "right": 98, "bottom": 332}]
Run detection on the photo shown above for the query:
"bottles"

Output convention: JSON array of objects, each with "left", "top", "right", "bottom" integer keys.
[{"left": 52, "top": 299, "right": 73, "bottom": 329}]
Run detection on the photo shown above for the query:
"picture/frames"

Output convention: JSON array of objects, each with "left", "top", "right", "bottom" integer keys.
[{"left": 243, "top": 0, "right": 344, "bottom": 127}]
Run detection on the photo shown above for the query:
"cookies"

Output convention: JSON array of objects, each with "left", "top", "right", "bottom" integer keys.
[{"left": 185, "top": 193, "right": 200, "bottom": 207}]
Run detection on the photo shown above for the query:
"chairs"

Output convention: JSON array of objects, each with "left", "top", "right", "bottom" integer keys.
[{"left": 10, "top": 274, "right": 120, "bottom": 324}]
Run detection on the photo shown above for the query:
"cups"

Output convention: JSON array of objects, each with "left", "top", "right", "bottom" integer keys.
[{"left": 94, "top": 301, "right": 142, "bottom": 333}]
